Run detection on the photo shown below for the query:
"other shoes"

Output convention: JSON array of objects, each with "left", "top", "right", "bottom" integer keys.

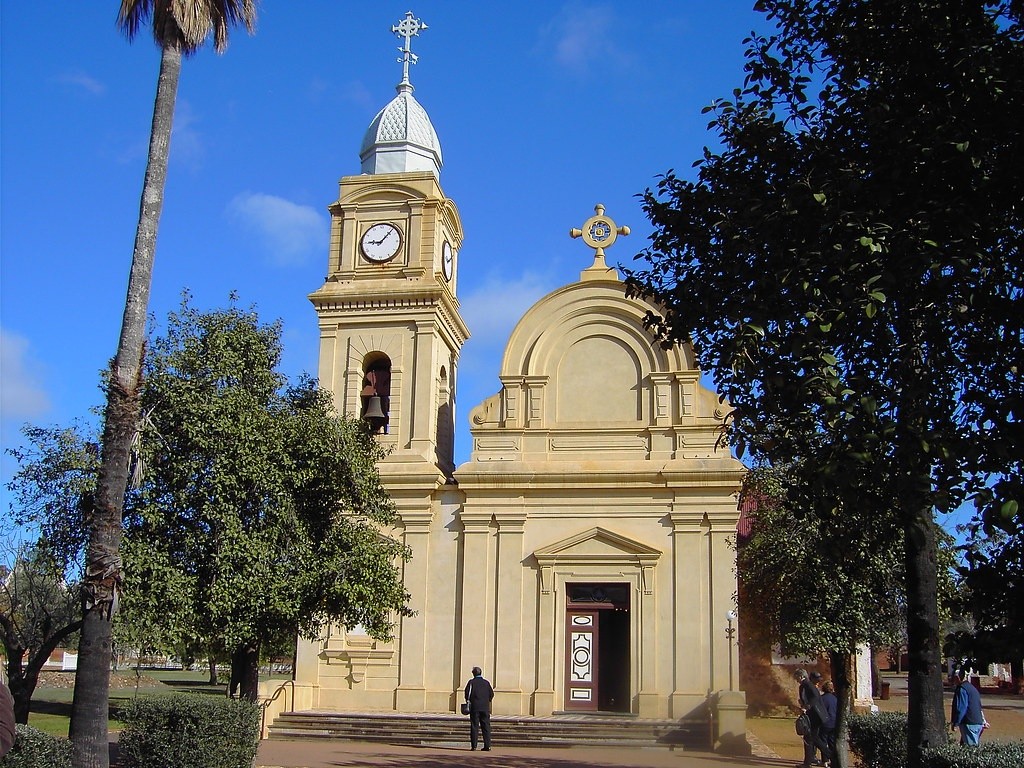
[
  {"left": 471, "top": 747, "right": 476, "bottom": 751},
  {"left": 481, "top": 747, "right": 491, "bottom": 751},
  {"left": 796, "top": 764, "right": 812, "bottom": 768}
]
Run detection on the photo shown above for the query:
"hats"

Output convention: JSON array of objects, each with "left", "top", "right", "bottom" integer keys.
[{"left": 810, "top": 672, "right": 823, "bottom": 681}]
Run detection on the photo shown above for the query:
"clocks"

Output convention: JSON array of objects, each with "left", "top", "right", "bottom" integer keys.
[
  {"left": 359, "top": 223, "right": 403, "bottom": 264},
  {"left": 443, "top": 241, "right": 453, "bottom": 282}
]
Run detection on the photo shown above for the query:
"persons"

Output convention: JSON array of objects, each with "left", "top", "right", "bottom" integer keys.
[
  {"left": 809, "top": 671, "right": 823, "bottom": 695},
  {"left": 794, "top": 667, "right": 835, "bottom": 768},
  {"left": 817, "top": 681, "right": 838, "bottom": 767},
  {"left": 950, "top": 669, "right": 990, "bottom": 747},
  {"left": 461, "top": 667, "right": 494, "bottom": 751},
  {"left": 0, "top": 683, "right": 16, "bottom": 759}
]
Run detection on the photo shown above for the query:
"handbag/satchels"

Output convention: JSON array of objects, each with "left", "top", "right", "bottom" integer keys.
[
  {"left": 796, "top": 713, "right": 811, "bottom": 735},
  {"left": 461, "top": 703, "right": 471, "bottom": 715}
]
